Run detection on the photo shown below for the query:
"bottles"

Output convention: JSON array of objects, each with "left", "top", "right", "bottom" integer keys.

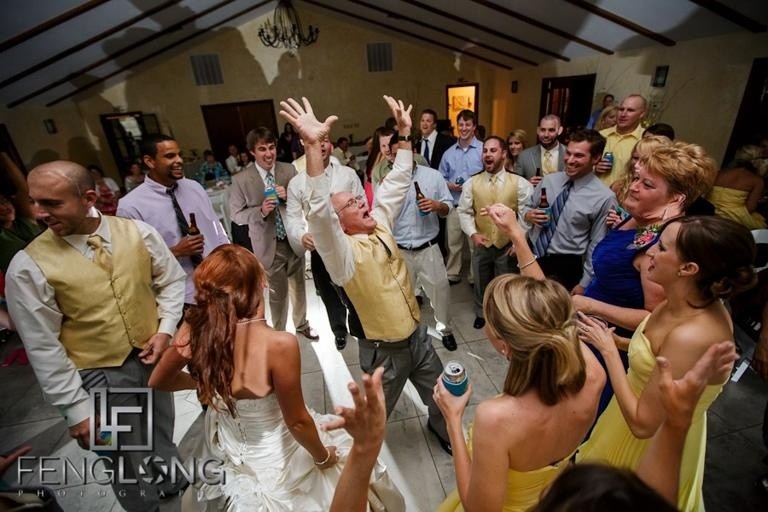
[
  {"left": 538, "top": 184, "right": 550, "bottom": 227},
  {"left": 533, "top": 167, "right": 540, "bottom": 189},
  {"left": 413, "top": 181, "right": 430, "bottom": 216},
  {"left": 184, "top": 213, "right": 202, "bottom": 259},
  {"left": 58, "top": 407, "right": 117, "bottom": 448}
]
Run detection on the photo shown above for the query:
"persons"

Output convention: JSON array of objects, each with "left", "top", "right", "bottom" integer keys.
[{"left": 1, "top": 94, "right": 768, "bottom": 512}]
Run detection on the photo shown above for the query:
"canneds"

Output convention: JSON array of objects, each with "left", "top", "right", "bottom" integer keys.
[
  {"left": 455, "top": 176, "right": 464, "bottom": 186},
  {"left": 263, "top": 187, "right": 279, "bottom": 207},
  {"left": 91, "top": 426, "right": 112, "bottom": 446},
  {"left": 604, "top": 152, "right": 613, "bottom": 172},
  {"left": 443, "top": 362, "right": 466, "bottom": 383}
]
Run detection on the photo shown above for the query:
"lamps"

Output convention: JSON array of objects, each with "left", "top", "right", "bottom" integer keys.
[{"left": 258, "top": 0, "right": 319, "bottom": 49}]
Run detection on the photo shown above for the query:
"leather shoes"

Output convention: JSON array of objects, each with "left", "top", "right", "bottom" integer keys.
[
  {"left": 334, "top": 335, "right": 347, "bottom": 350},
  {"left": 473, "top": 316, "right": 486, "bottom": 329},
  {"left": 298, "top": 327, "right": 320, "bottom": 340},
  {"left": 441, "top": 334, "right": 458, "bottom": 351}
]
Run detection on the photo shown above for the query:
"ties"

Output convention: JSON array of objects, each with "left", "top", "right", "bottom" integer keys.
[
  {"left": 532, "top": 179, "right": 574, "bottom": 260},
  {"left": 489, "top": 174, "right": 500, "bottom": 206},
  {"left": 265, "top": 171, "right": 288, "bottom": 241},
  {"left": 86, "top": 235, "right": 114, "bottom": 272},
  {"left": 422, "top": 137, "right": 431, "bottom": 164},
  {"left": 164, "top": 184, "right": 204, "bottom": 269},
  {"left": 542, "top": 150, "right": 555, "bottom": 176}
]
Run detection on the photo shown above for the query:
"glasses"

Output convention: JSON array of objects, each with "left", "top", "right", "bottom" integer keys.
[{"left": 336, "top": 194, "right": 363, "bottom": 216}]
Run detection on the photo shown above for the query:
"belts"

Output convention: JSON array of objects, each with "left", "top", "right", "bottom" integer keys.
[{"left": 396, "top": 238, "right": 436, "bottom": 252}]
